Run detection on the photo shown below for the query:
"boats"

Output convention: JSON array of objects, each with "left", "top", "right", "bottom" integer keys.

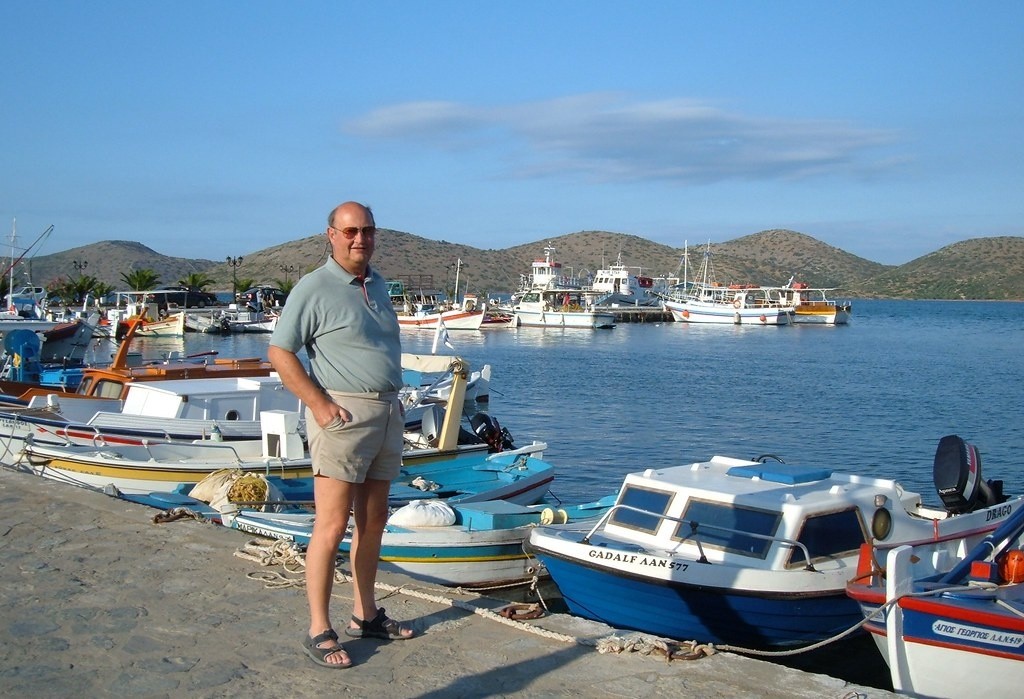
[
  {"left": 846, "top": 502, "right": 1024, "bottom": 699},
  {"left": 0, "top": 237, "right": 853, "bottom": 594},
  {"left": 523, "top": 454, "right": 1024, "bottom": 655}
]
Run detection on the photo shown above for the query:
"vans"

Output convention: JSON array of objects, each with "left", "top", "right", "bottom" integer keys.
[{"left": 4, "top": 287, "right": 50, "bottom": 306}]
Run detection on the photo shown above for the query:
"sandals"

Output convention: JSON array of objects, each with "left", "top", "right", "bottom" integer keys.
[
  {"left": 300, "top": 629, "right": 352, "bottom": 669},
  {"left": 343, "top": 607, "right": 413, "bottom": 639}
]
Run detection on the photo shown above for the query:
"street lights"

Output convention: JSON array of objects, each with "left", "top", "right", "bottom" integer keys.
[
  {"left": 225, "top": 254, "right": 244, "bottom": 304},
  {"left": 279, "top": 263, "right": 295, "bottom": 285}
]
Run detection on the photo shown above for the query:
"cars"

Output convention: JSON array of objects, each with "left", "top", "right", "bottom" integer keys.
[
  {"left": 236, "top": 286, "right": 286, "bottom": 306},
  {"left": 152, "top": 286, "right": 218, "bottom": 309}
]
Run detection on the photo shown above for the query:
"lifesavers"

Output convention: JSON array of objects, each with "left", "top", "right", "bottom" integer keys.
[
  {"left": 733, "top": 300, "right": 741, "bottom": 309},
  {"left": 563, "top": 276, "right": 569, "bottom": 283}
]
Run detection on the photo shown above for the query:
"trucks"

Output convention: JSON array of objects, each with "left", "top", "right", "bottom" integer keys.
[{"left": 384, "top": 280, "right": 443, "bottom": 302}]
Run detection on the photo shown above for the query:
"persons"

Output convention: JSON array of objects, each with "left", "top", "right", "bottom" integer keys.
[{"left": 267, "top": 201, "right": 412, "bottom": 668}]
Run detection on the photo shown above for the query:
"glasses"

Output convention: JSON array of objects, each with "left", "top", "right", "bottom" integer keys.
[{"left": 331, "top": 226, "right": 377, "bottom": 239}]
